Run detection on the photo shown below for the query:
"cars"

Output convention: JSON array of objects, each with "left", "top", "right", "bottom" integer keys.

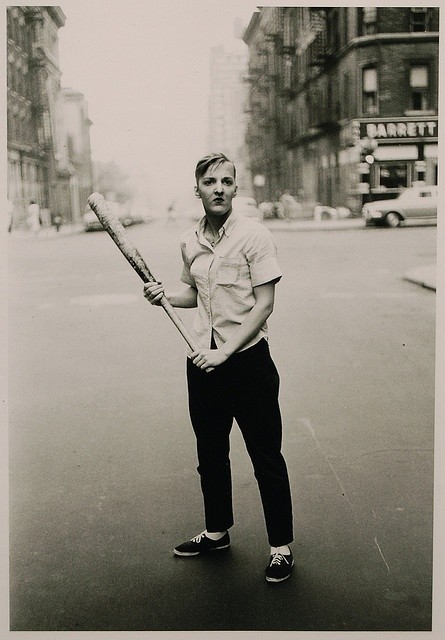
[{"left": 84, "top": 202, "right": 132, "bottom": 231}]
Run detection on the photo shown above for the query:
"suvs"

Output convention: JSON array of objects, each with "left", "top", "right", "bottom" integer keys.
[{"left": 361, "top": 181, "right": 440, "bottom": 228}]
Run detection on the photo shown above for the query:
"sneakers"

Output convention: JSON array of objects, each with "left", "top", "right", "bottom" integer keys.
[
  {"left": 265, "top": 549, "right": 294, "bottom": 584},
  {"left": 173, "top": 530, "right": 230, "bottom": 555}
]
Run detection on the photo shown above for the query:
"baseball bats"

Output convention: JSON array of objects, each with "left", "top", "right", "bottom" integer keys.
[{"left": 86, "top": 191, "right": 215, "bottom": 376}]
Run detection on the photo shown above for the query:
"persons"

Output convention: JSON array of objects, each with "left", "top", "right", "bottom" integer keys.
[
  {"left": 27, "top": 200, "right": 40, "bottom": 237},
  {"left": 143, "top": 153, "right": 295, "bottom": 584}
]
